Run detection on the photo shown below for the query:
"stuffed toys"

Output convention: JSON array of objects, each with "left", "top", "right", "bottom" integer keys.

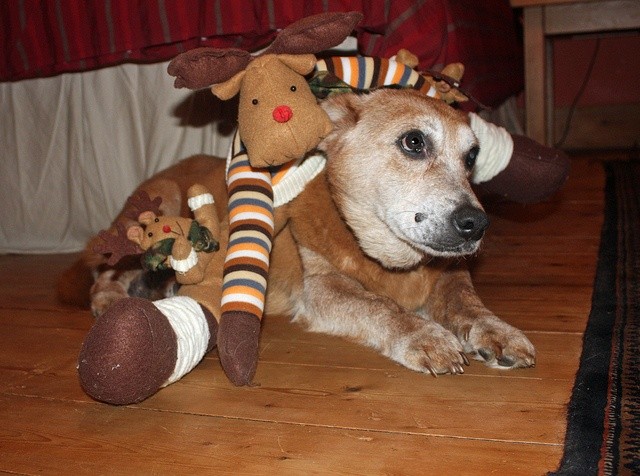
[{"left": 79, "top": 11, "right": 572, "bottom": 405}]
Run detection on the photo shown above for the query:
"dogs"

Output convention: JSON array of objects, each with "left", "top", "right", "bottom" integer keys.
[{"left": 54, "top": 85, "right": 536, "bottom": 378}]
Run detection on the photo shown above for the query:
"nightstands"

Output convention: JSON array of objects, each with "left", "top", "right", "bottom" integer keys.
[{"left": 510, "top": 0, "right": 640, "bottom": 149}]
